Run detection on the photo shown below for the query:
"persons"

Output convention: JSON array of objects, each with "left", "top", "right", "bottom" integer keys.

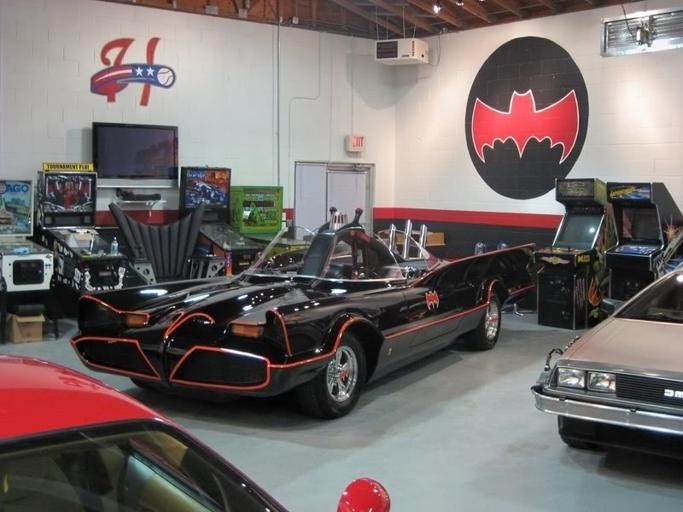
[
  {"left": 48, "top": 181, "right": 64, "bottom": 209},
  {"left": 73, "top": 181, "right": 88, "bottom": 207},
  {"left": 61, "top": 182, "right": 79, "bottom": 209}
]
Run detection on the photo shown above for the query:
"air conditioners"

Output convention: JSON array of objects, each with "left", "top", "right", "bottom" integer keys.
[{"left": 373, "top": 4, "right": 429, "bottom": 65}]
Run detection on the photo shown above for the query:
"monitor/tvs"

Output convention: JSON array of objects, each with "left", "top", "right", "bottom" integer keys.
[
  {"left": 622, "top": 206, "right": 661, "bottom": 240},
  {"left": 554, "top": 214, "right": 602, "bottom": 251},
  {"left": 92, "top": 122, "right": 178, "bottom": 189}
]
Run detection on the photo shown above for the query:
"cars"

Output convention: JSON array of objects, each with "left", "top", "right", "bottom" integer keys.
[
  {"left": 531, "top": 261, "right": 683, "bottom": 462},
  {"left": 0, "top": 354, "right": 388, "bottom": 512},
  {"left": 68, "top": 207, "right": 536, "bottom": 419}
]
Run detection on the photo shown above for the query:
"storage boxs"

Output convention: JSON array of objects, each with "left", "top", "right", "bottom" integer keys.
[{"left": 5, "top": 312, "right": 46, "bottom": 343}]
